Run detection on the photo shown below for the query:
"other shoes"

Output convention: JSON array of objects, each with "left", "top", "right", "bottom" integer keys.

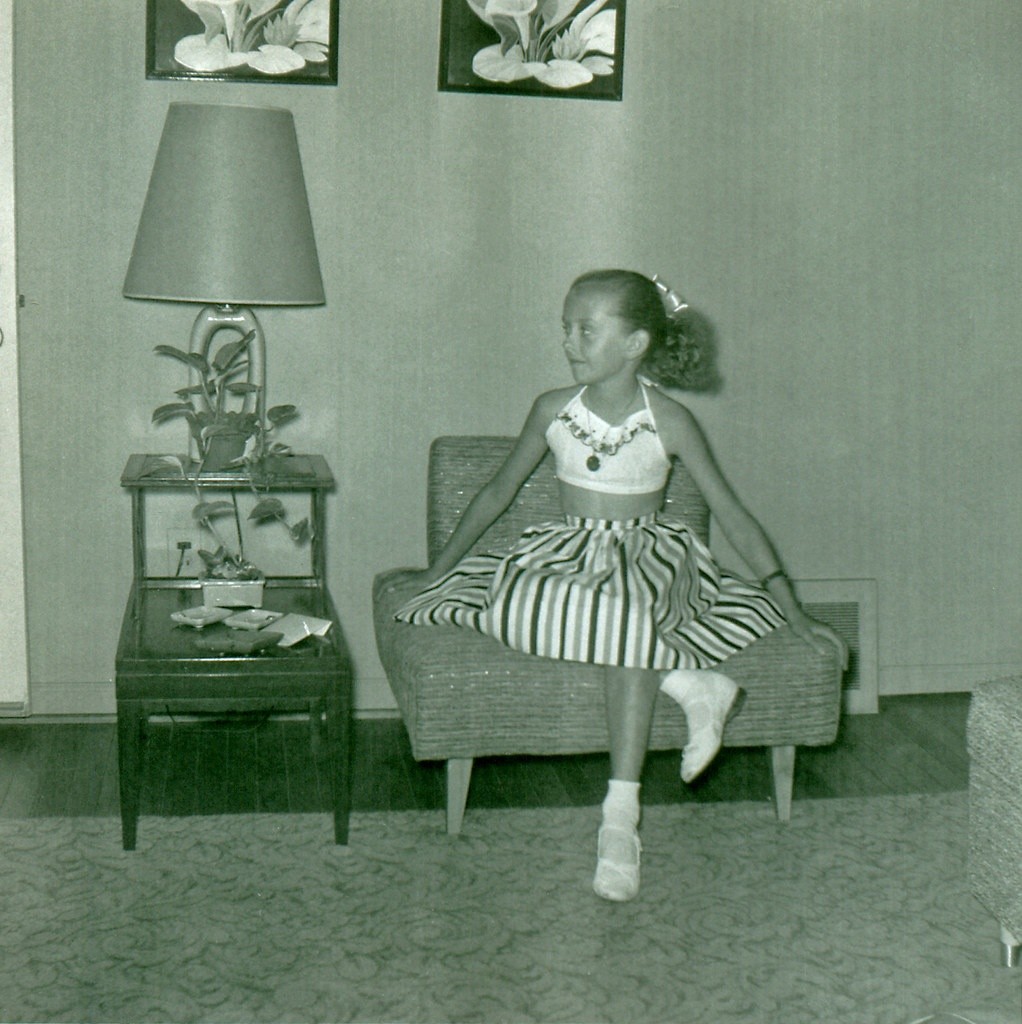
[
  {"left": 595, "top": 827, "right": 642, "bottom": 903},
  {"left": 680, "top": 669, "right": 740, "bottom": 786}
]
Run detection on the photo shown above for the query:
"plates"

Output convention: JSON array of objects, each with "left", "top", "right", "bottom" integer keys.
[
  {"left": 224, "top": 609, "right": 282, "bottom": 630},
  {"left": 196, "top": 630, "right": 284, "bottom": 653},
  {"left": 171, "top": 605, "right": 233, "bottom": 627}
]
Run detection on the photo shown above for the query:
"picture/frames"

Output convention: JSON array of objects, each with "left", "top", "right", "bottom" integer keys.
[
  {"left": 437, "top": 0, "right": 625, "bottom": 100},
  {"left": 145, "top": 0, "right": 338, "bottom": 85}
]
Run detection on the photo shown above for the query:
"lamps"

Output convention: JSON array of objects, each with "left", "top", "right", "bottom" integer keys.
[{"left": 121, "top": 103, "right": 328, "bottom": 468}]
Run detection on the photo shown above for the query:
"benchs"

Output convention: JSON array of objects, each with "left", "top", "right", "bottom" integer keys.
[{"left": 373, "top": 437, "right": 841, "bottom": 840}]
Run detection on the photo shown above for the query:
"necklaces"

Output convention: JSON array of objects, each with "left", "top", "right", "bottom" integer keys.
[{"left": 586, "top": 375, "right": 641, "bottom": 471}]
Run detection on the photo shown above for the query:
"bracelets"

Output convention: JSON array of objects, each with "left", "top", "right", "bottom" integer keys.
[{"left": 761, "top": 570, "right": 787, "bottom": 589}]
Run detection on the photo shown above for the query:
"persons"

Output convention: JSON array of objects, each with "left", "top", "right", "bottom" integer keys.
[{"left": 373, "top": 269, "right": 850, "bottom": 902}]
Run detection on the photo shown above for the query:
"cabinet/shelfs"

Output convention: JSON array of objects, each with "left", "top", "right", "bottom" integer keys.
[{"left": 115, "top": 451, "right": 356, "bottom": 850}]
